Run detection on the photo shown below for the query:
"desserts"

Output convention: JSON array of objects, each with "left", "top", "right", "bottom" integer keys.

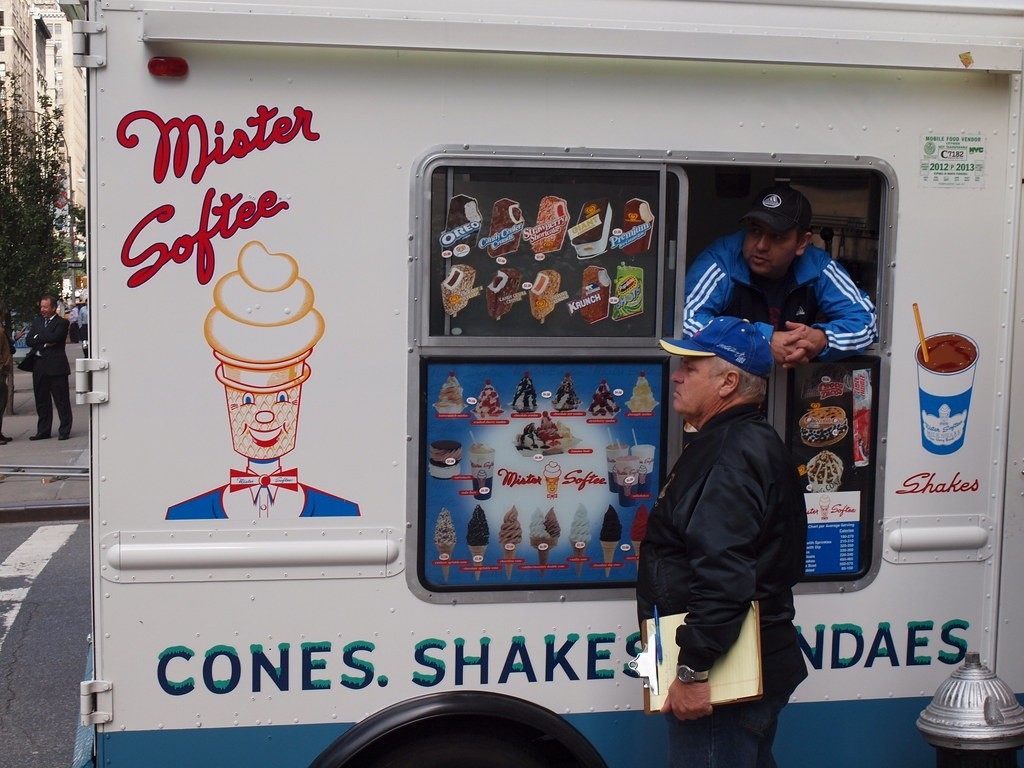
[
  {"left": 431, "top": 440, "right": 462, "bottom": 463},
  {"left": 429, "top": 458, "right": 463, "bottom": 480},
  {"left": 799, "top": 406, "right": 849, "bottom": 447}
]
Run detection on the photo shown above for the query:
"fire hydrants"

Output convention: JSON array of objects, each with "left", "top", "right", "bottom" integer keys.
[{"left": 912, "top": 653, "right": 1023, "bottom": 768}]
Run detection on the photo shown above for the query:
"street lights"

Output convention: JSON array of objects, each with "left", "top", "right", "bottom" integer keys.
[{"left": 18, "top": 108, "right": 76, "bottom": 305}]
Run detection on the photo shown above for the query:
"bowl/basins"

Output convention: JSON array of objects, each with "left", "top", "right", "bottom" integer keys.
[
  {"left": 548, "top": 401, "right": 584, "bottom": 411},
  {"left": 432, "top": 403, "right": 469, "bottom": 414},
  {"left": 625, "top": 400, "right": 660, "bottom": 412},
  {"left": 470, "top": 409, "right": 506, "bottom": 420},
  {"left": 507, "top": 402, "right": 540, "bottom": 412},
  {"left": 586, "top": 408, "right": 622, "bottom": 419}
]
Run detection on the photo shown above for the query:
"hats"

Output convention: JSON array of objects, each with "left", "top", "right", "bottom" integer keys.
[
  {"left": 739, "top": 185, "right": 812, "bottom": 231},
  {"left": 658, "top": 315, "right": 773, "bottom": 379}
]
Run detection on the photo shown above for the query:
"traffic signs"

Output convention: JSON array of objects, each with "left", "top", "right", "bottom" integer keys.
[{"left": 63, "top": 261, "right": 82, "bottom": 268}]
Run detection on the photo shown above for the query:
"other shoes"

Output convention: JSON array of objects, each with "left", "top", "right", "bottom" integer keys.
[
  {"left": 0, "top": 434, "right": 12, "bottom": 441},
  {"left": 29, "top": 434, "right": 51, "bottom": 440},
  {"left": 0, "top": 441, "right": 7, "bottom": 445},
  {"left": 59, "top": 434, "right": 69, "bottom": 439}
]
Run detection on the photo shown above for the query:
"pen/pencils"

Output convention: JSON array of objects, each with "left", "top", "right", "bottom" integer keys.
[{"left": 654, "top": 605, "right": 663, "bottom": 665}]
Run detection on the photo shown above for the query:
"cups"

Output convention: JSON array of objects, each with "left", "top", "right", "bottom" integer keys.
[
  {"left": 615, "top": 456, "right": 641, "bottom": 508},
  {"left": 606, "top": 445, "right": 629, "bottom": 493},
  {"left": 469, "top": 449, "right": 496, "bottom": 501},
  {"left": 631, "top": 444, "right": 656, "bottom": 494}
]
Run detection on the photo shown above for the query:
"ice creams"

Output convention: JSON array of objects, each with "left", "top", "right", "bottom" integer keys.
[
  {"left": 431, "top": 371, "right": 662, "bottom": 580},
  {"left": 807, "top": 450, "right": 844, "bottom": 493}
]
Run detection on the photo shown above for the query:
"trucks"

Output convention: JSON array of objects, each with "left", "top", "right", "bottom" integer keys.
[{"left": 67, "top": 0, "right": 1024, "bottom": 768}]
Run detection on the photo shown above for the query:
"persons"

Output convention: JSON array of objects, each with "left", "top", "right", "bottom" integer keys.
[
  {"left": 684, "top": 180, "right": 879, "bottom": 373},
  {"left": 636, "top": 316, "right": 820, "bottom": 768},
  {"left": 17, "top": 295, "right": 74, "bottom": 441},
  {"left": 0, "top": 300, "right": 28, "bottom": 446},
  {"left": 65, "top": 298, "right": 88, "bottom": 358}
]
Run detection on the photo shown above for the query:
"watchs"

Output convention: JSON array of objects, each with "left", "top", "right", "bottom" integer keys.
[{"left": 675, "top": 665, "right": 710, "bottom": 683}]
